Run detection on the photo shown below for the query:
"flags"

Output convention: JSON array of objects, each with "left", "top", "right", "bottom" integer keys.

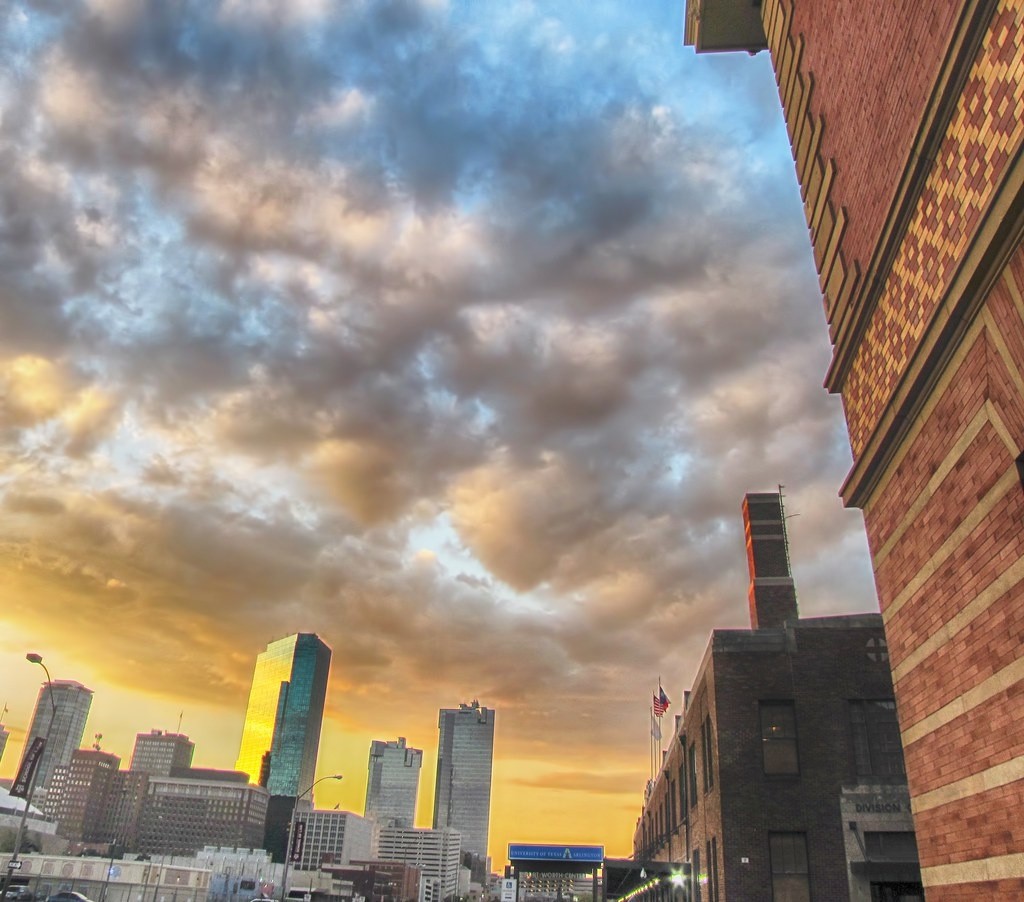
[
  {"left": 654, "top": 696, "right": 663, "bottom": 718},
  {"left": 652, "top": 718, "right": 663, "bottom": 741},
  {"left": 660, "top": 687, "right": 671, "bottom": 713}
]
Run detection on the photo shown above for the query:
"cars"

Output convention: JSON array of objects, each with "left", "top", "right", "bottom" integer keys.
[
  {"left": 45, "top": 891, "right": 93, "bottom": 902},
  {"left": 0, "top": 884, "right": 34, "bottom": 902}
]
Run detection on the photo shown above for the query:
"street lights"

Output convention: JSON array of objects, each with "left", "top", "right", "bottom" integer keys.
[
  {"left": 0, "top": 652, "right": 59, "bottom": 902},
  {"left": 280, "top": 774, "right": 344, "bottom": 902}
]
